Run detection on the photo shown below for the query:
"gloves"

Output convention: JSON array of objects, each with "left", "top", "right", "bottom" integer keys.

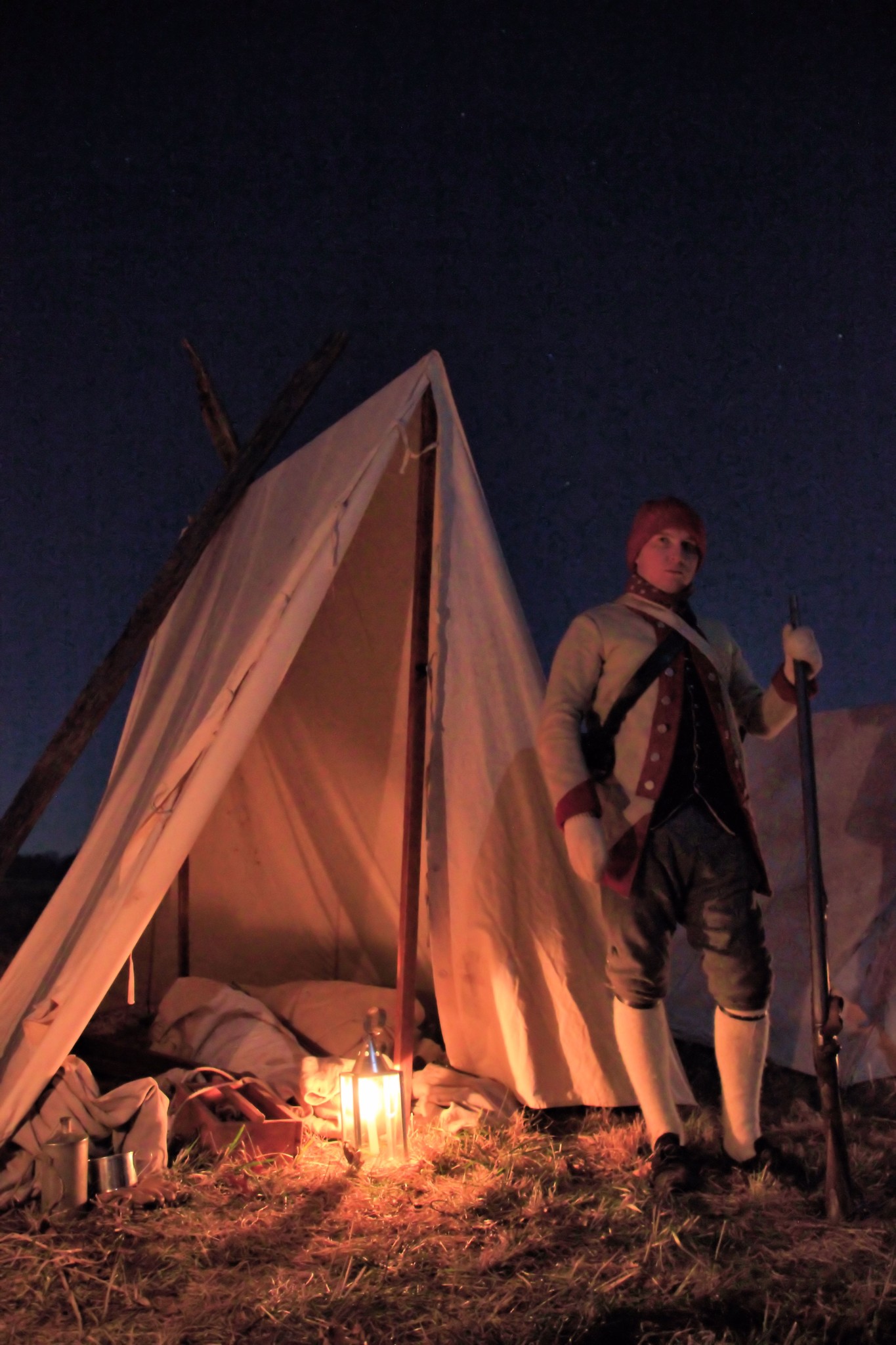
[
  {"left": 781, "top": 624, "right": 823, "bottom": 685},
  {"left": 562, "top": 813, "right": 606, "bottom": 881}
]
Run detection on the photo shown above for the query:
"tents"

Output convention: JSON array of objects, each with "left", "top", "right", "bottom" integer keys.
[
  {"left": 0, "top": 347, "right": 694, "bottom": 1109},
  {"left": 664, "top": 704, "right": 896, "bottom": 1087}
]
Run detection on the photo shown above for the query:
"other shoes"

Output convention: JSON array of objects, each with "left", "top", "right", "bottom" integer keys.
[
  {"left": 648, "top": 1134, "right": 691, "bottom": 1184},
  {"left": 718, "top": 1134, "right": 822, "bottom": 1193}
]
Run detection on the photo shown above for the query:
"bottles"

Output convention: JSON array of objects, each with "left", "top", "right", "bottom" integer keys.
[
  {"left": 365, "top": 1007, "right": 395, "bottom": 1062},
  {"left": 39, "top": 1116, "right": 89, "bottom": 1215}
]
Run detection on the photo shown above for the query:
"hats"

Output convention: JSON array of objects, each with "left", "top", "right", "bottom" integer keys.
[{"left": 626, "top": 495, "right": 707, "bottom": 572}]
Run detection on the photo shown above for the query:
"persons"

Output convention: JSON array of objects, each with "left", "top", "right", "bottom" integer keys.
[{"left": 537, "top": 499, "right": 823, "bottom": 1211}]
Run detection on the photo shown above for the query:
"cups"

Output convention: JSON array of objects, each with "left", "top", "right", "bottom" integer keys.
[{"left": 88, "top": 1148, "right": 153, "bottom": 1198}]
